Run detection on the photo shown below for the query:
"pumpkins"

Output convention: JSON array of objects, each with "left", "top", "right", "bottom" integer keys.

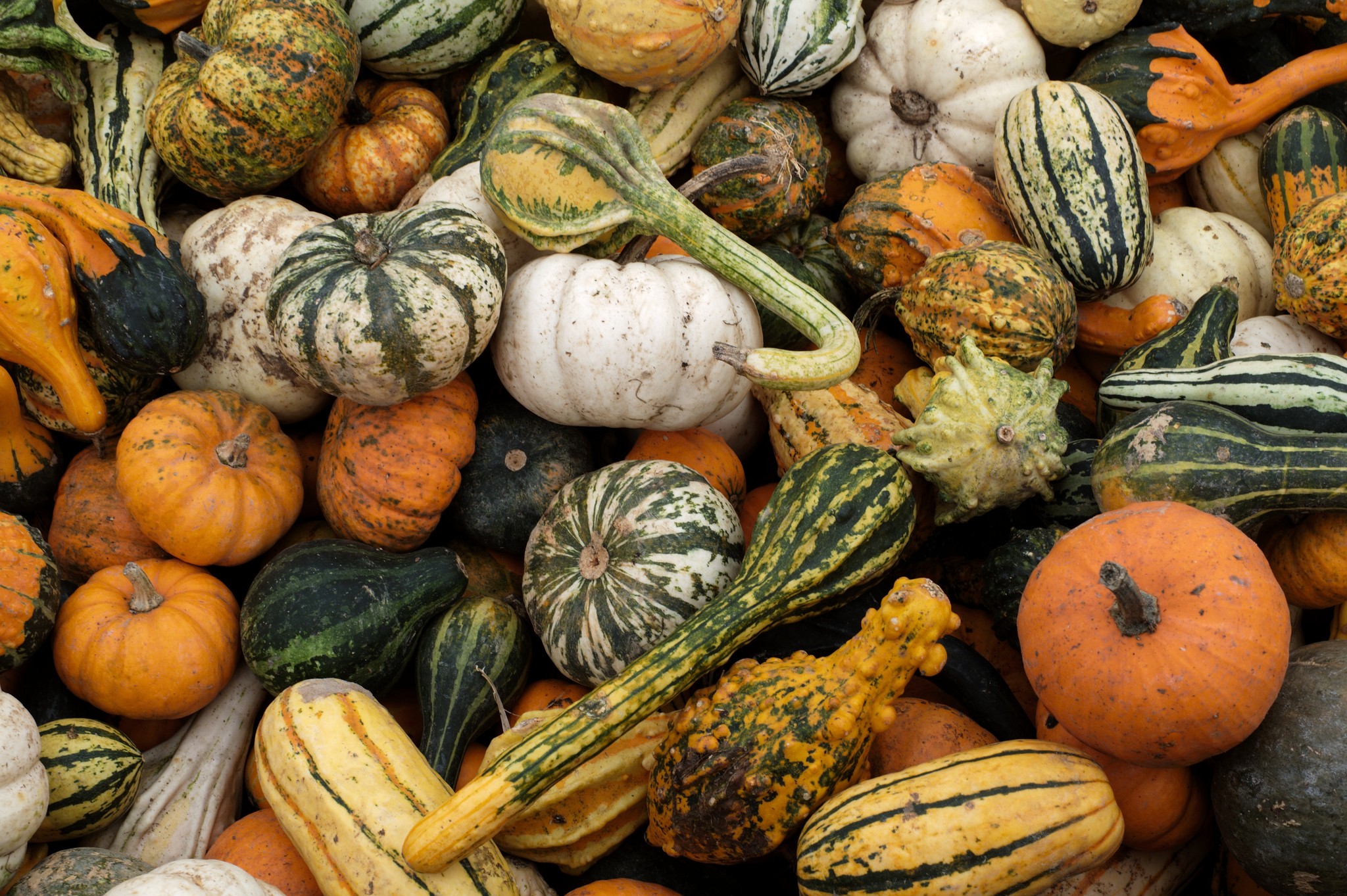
[{"left": 0, "top": 0, "right": 1347, "bottom": 896}]
